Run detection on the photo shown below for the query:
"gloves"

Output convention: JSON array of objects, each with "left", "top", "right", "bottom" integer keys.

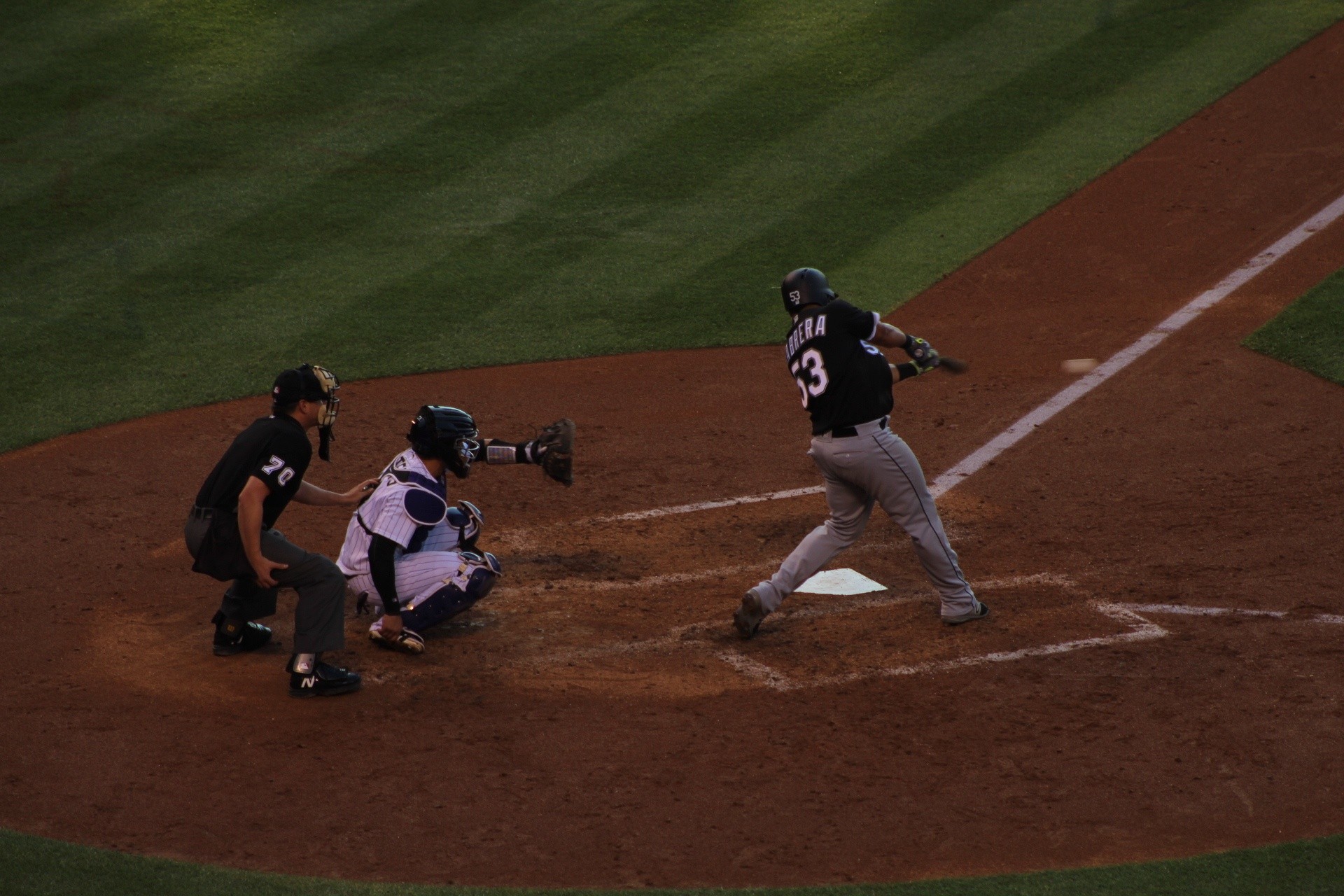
[
  {"left": 906, "top": 335, "right": 939, "bottom": 367},
  {"left": 908, "top": 358, "right": 937, "bottom": 374}
]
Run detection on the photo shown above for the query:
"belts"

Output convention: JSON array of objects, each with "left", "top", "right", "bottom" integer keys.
[
  {"left": 190, "top": 504, "right": 214, "bottom": 520},
  {"left": 832, "top": 418, "right": 886, "bottom": 439}
]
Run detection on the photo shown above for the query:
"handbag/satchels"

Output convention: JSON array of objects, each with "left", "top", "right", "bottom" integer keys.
[{"left": 190, "top": 508, "right": 243, "bottom": 582}]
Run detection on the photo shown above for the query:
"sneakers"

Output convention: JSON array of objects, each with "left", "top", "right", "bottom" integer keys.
[
  {"left": 941, "top": 600, "right": 989, "bottom": 624},
  {"left": 733, "top": 588, "right": 768, "bottom": 639},
  {"left": 291, "top": 659, "right": 361, "bottom": 699},
  {"left": 370, "top": 619, "right": 426, "bottom": 653},
  {"left": 213, "top": 621, "right": 272, "bottom": 657}
]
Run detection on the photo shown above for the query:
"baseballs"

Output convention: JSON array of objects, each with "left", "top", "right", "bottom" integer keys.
[{"left": 1063, "top": 359, "right": 1097, "bottom": 372}]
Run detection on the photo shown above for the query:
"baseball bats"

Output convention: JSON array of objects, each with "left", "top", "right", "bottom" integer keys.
[{"left": 914, "top": 349, "right": 968, "bottom": 374}]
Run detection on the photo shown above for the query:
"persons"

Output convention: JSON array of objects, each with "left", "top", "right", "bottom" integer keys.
[
  {"left": 335, "top": 405, "right": 575, "bottom": 652},
  {"left": 734, "top": 267, "right": 990, "bottom": 638},
  {"left": 184, "top": 364, "right": 380, "bottom": 698}
]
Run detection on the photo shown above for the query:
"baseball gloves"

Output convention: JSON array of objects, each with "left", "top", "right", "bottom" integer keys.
[{"left": 537, "top": 418, "right": 572, "bottom": 488}]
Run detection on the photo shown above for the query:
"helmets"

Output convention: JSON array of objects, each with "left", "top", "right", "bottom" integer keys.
[
  {"left": 407, "top": 404, "right": 481, "bottom": 479},
  {"left": 271, "top": 364, "right": 330, "bottom": 406},
  {"left": 782, "top": 267, "right": 839, "bottom": 316}
]
